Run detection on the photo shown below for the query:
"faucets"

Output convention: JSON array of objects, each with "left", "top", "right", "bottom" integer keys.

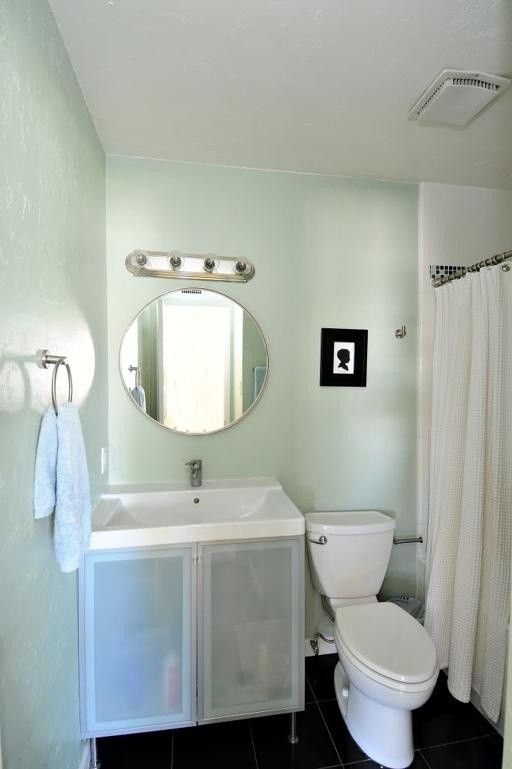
[{"left": 183, "top": 459, "right": 202, "bottom": 487}]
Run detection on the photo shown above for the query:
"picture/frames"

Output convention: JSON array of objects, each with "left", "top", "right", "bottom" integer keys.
[{"left": 320, "top": 328, "right": 368, "bottom": 387}]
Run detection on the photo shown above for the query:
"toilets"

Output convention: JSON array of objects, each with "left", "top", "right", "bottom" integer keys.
[{"left": 305, "top": 511, "right": 441, "bottom": 769}]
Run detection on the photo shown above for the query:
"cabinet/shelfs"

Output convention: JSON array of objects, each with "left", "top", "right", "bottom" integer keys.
[{"left": 84, "top": 537, "right": 306, "bottom": 726}]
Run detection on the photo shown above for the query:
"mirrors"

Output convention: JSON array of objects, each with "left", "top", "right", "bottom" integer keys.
[{"left": 118, "top": 287, "right": 270, "bottom": 439}]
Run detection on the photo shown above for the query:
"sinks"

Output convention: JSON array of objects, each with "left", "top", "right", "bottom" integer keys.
[{"left": 84, "top": 478, "right": 306, "bottom": 550}]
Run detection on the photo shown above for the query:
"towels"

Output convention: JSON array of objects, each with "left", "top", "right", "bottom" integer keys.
[
  {"left": 36, "top": 404, "right": 92, "bottom": 574},
  {"left": 130, "top": 386, "right": 146, "bottom": 413}
]
